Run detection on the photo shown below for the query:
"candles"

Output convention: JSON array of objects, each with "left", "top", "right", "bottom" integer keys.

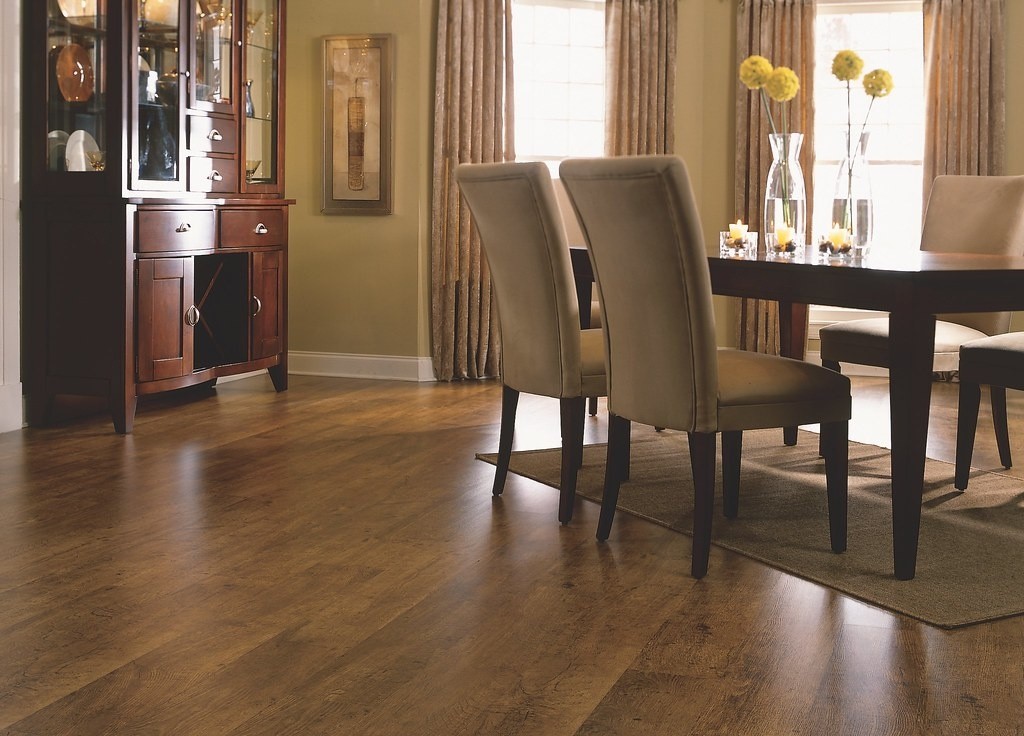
[
  {"left": 776, "top": 222, "right": 795, "bottom": 249},
  {"left": 729, "top": 220, "right": 748, "bottom": 243},
  {"left": 828, "top": 223, "right": 848, "bottom": 249}
]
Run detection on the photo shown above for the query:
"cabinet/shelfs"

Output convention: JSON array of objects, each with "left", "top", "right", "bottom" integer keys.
[{"left": 19, "top": 0, "right": 299, "bottom": 433}]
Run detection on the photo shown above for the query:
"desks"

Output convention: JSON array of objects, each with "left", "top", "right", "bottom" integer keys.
[{"left": 571, "top": 243, "right": 1024, "bottom": 580}]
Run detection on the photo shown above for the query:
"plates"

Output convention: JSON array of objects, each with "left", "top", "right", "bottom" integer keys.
[
  {"left": 55, "top": 43, "right": 94, "bottom": 102},
  {"left": 138, "top": 55, "right": 151, "bottom": 72},
  {"left": 48, "top": 130, "right": 70, "bottom": 171},
  {"left": 66, "top": 130, "right": 102, "bottom": 172},
  {"left": 58, "top": 0, "right": 96, "bottom": 28}
]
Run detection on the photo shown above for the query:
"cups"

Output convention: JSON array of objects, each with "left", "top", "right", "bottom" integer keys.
[
  {"left": 719, "top": 231, "right": 758, "bottom": 260},
  {"left": 139, "top": 70, "right": 158, "bottom": 105}
]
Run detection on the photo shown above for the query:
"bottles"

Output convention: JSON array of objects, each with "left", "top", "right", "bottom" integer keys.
[{"left": 246, "top": 80, "right": 255, "bottom": 117}]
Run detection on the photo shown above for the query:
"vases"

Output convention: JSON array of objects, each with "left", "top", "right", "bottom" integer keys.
[
  {"left": 764, "top": 133, "right": 807, "bottom": 252},
  {"left": 831, "top": 127, "right": 874, "bottom": 253}
]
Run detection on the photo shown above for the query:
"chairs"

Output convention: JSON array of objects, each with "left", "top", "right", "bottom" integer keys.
[
  {"left": 560, "top": 156, "right": 855, "bottom": 582},
  {"left": 954, "top": 330, "right": 1024, "bottom": 494},
  {"left": 455, "top": 158, "right": 697, "bottom": 530},
  {"left": 816, "top": 174, "right": 1024, "bottom": 471}
]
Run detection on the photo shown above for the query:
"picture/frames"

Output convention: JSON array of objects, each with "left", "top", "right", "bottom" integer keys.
[{"left": 320, "top": 32, "right": 393, "bottom": 216}]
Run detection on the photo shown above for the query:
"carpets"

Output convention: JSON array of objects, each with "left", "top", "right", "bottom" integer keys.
[{"left": 472, "top": 427, "right": 1024, "bottom": 630}]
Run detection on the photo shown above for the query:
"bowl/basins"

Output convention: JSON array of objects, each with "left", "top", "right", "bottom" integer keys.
[{"left": 247, "top": 160, "right": 261, "bottom": 182}]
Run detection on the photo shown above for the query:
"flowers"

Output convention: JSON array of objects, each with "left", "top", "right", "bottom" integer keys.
[
  {"left": 738, "top": 56, "right": 799, "bottom": 226},
  {"left": 831, "top": 49, "right": 894, "bottom": 235}
]
